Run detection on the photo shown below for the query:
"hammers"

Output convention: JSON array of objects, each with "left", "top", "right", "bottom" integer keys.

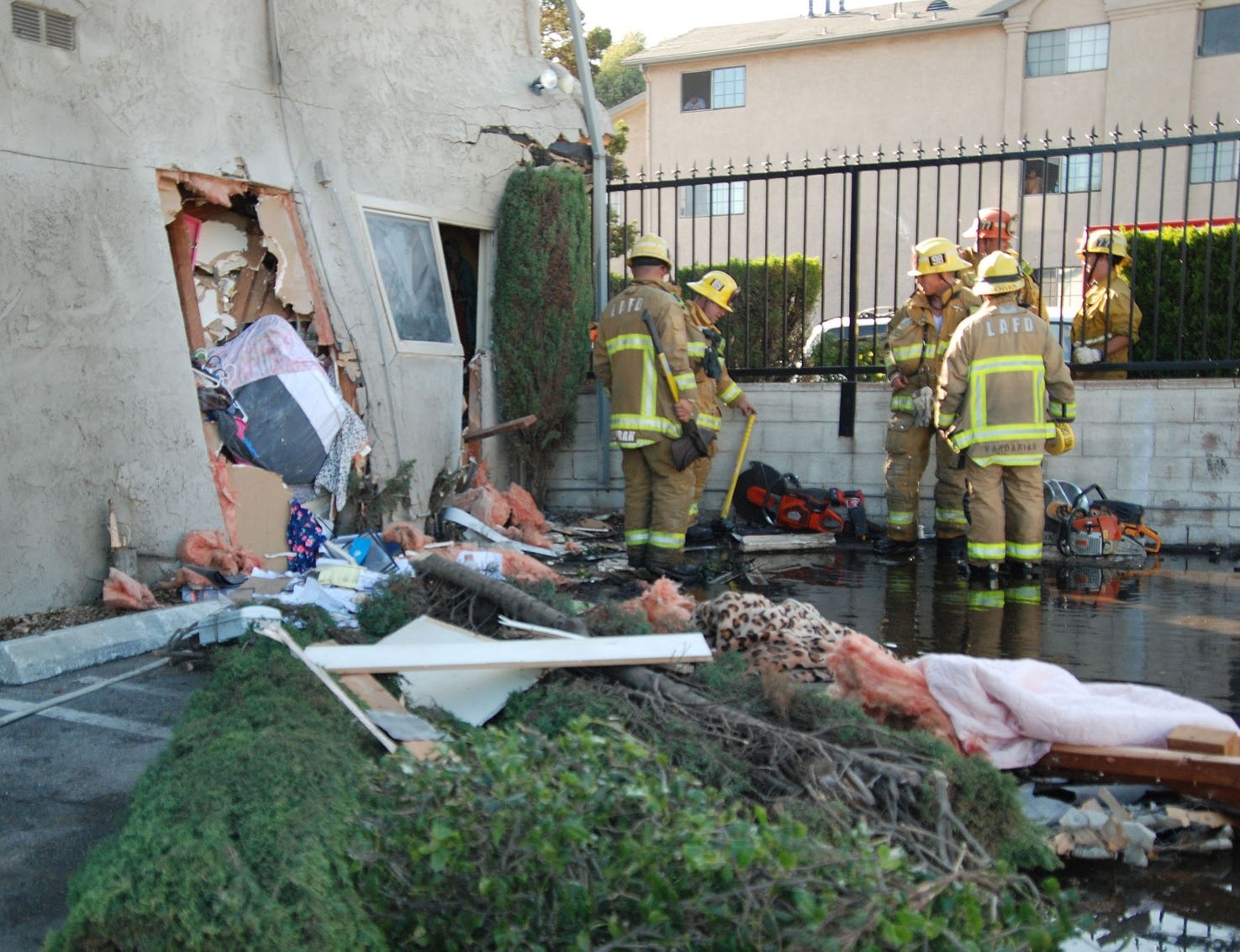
[{"left": 711, "top": 414, "right": 756, "bottom": 533}]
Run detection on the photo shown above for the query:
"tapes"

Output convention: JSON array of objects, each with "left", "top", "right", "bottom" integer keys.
[{"left": 1045, "top": 423, "right": 1074, "bottom": 454}]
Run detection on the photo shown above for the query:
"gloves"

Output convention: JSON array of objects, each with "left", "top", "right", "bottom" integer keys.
[
  {"left": 914, "top": 386, "right": 933, "bottom": 428},
  {"left": 1072, "top": 346, "right": 1101, "bottom": 364}
]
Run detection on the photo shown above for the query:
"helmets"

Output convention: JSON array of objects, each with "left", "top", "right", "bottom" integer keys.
[
  {"left": 962, "top": 208, "right": 1013, "bottom": 238},
  {"left": 686, "top": 270, "right": 742, "bottom": 313},
  {"left": 907, "top": 237, "right": 972, "bottom": 276},
  {"left": 973, "top": 250, "right": 1025, "bottom": 295},
  {"left": 626, "top": 233, "right": 672, "bottom": 274},
  {"left": 1075, "top": 229, "right": 1132, "bottom": 267}
]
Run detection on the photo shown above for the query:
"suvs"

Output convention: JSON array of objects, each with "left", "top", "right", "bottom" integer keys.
[{"left": 790, "top": 307, "right": 1076, "bottom": 383}]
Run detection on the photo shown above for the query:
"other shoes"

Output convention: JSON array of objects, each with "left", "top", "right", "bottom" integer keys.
[
  {"left": 999, "top": 556, "right": 1043, "bottom": 572},
  {"left": 1002, "top": 573, "right": 1042, "bottom": 588},
  {"left": 626, "top": 546, "right": 648, "bottom": 566},
  {"left": 967, "top": 559, "right": 1005, "bottom": 578},
  {"left": 936, "top": 533, "right": 967, "bottom": 549},
  {"left": 870, "top": 538, "right": 920, "bottom": 553},
  {"left": 968, "top": 577, "right": 1005, "bottom": 591},
  {"left": 685, "top": 525, "right": 714, "bottom": 541},
  {"left": 936, "top": 550, "right": 964, "bottom": 560},
  {"left": 872, "top": 553, "right": 920, "bottom": 565},
  {"left": 649, "top": 558, "right": 700, "bottom": 581}
]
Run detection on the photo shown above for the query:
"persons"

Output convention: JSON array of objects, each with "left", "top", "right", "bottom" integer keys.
[
  {"left": 959, "top": 207, "right": 1050, "bottom": 326},
  {"left": 873, "top": 237, "right": 986, "bottom": 552},
  {"left": 680, "top": 270, "right": 758, "bottom": 542},
  {"left": 593, "top": 233, "right": 704, "bottom": 580},
  {"left": 934, "top": 250, "right": 1078, "bottom": 575},
  {"left": 1070, "top": 229, "right": 1142, "bottom": 380}
]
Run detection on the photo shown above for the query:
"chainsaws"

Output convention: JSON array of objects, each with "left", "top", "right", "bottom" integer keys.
[
  {"left": 1043, "top": 479, "right": 1161, "bottom": 558},
  {"left": 1045, "top": 556, "right": 1161, "bottom": 609},
  {"left": 733, "top": 461, "right": 869, "bottom": 539}
]
